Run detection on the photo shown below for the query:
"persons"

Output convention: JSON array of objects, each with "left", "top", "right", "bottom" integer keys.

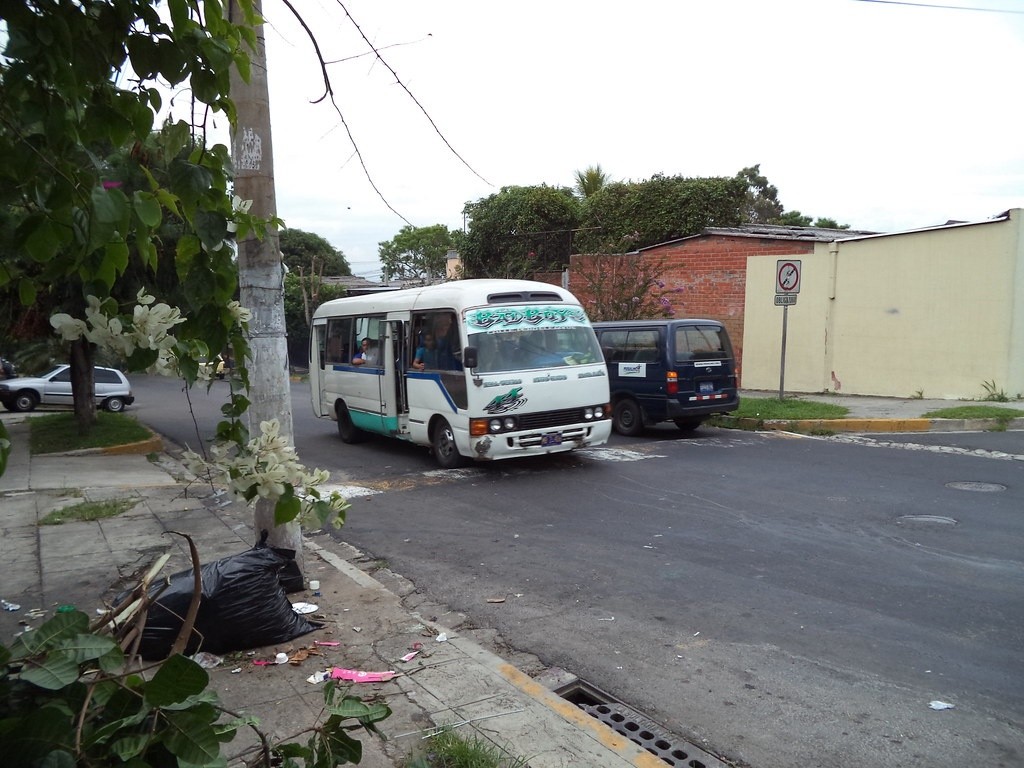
[
  {"left": 412, "top": 332, "right": 437, "bottom": 370},
  {"left": 351, "top": 337, "right": 373, "bottom": 365}
]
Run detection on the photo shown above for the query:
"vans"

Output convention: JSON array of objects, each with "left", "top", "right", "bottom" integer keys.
[{"left": 526, "top": 319, "right": 741, "bottom": 437}]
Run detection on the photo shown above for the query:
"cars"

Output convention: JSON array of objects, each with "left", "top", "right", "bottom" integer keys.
[
  {"left": 199, "top": 354, "right": 225, "bottom": 381},
  {"left": 1, "top": 364, "right": 135, "bottom": 412}
]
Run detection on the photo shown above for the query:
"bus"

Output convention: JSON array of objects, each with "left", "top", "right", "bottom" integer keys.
[{"left": 308, "top": 283, "right": 613, "bottom": 472}]
